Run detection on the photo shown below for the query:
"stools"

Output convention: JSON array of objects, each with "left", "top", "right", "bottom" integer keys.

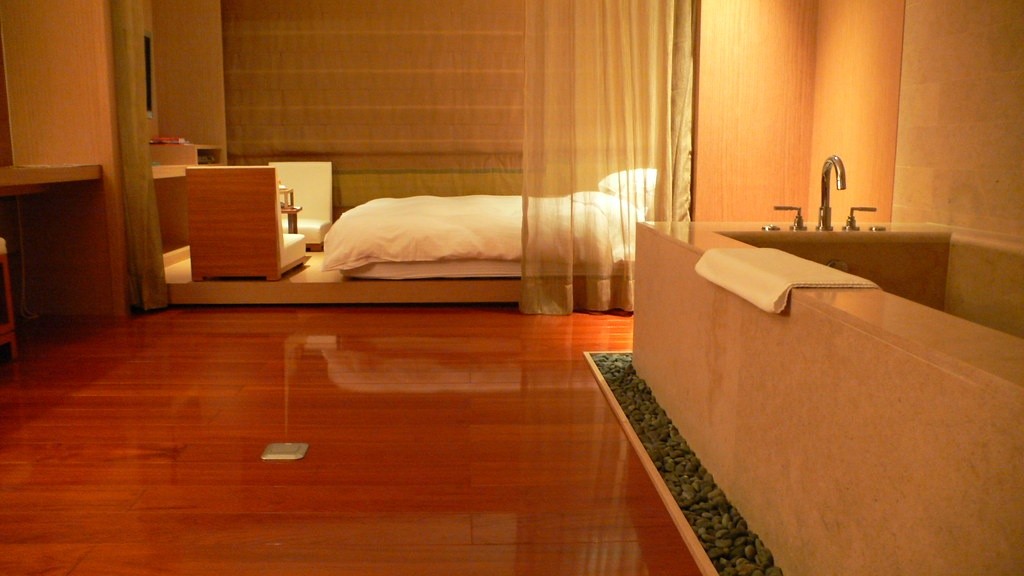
[{"left": 0, "top": 238, "right": 18, "bottom": 333}]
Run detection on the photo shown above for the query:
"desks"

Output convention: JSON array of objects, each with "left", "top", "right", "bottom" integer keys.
[{"left": 281, "top": 207, "right": 303, "bottom": 234}]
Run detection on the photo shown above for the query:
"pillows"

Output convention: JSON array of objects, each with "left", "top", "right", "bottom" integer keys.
[{"left": 597, "top": 168, "right": 658, "bottom": 209}]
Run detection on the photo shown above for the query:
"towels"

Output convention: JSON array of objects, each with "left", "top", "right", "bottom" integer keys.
[{"left": 694, "top": 248, "right": 881, "bottom": 314}]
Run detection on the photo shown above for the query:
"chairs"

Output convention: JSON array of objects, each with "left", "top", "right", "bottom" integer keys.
[
  {"left": 267, "top": 161, "right": 333, "bottom": 252},
  {"left": 184, "top": 165, "right": 307, "bottom": 280}
]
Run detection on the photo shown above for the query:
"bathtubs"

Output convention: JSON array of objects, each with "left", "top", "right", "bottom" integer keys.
[{"left": 631, "top": 222, "right": 1024, "bottom": 576}]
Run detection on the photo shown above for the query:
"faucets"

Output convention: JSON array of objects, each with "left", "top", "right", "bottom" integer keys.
[{"left": 815, "top": 154, "right": 846, "bottom": 231}]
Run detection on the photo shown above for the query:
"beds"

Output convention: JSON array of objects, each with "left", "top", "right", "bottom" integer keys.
[{"left": 319, "top": 191, "right": 646, "bottom": 280}]
